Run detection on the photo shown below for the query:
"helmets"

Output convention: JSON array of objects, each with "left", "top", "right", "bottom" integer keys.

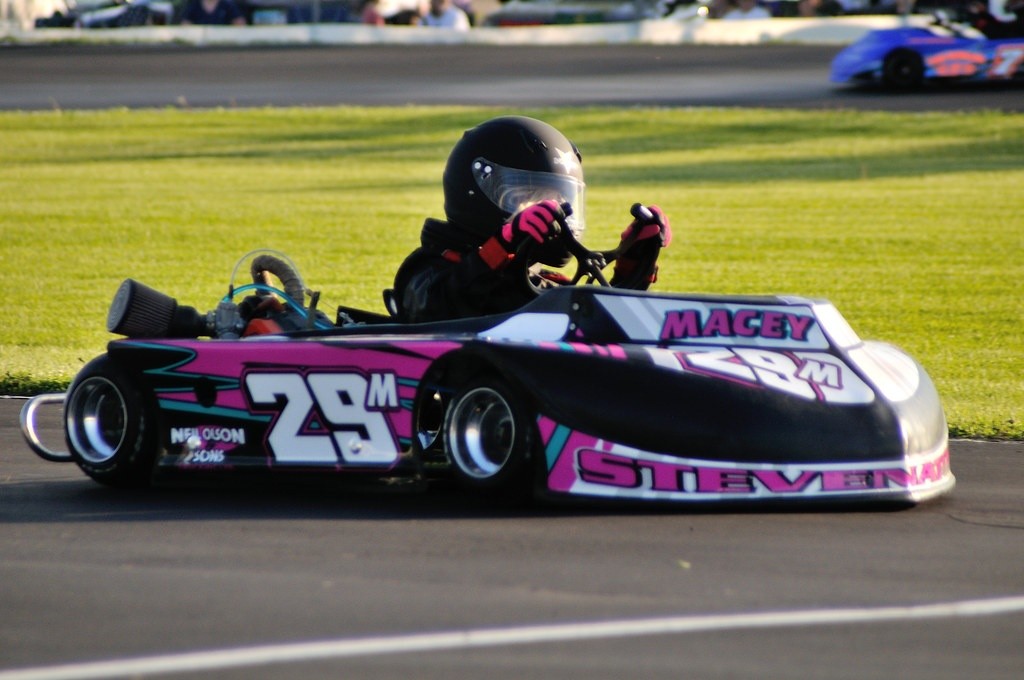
[{"left": 444, "top": 115, "right": 586, "bottom": 268}]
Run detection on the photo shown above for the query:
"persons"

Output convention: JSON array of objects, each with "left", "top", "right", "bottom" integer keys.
[
  {"left": 664, "top": 0, "right": 1024, "bottom": 39},
  {"left": 363, "top": 0, "right": 472, "bottom": 28},
  {"left": 180, "top": 0, "right": 246, "bottom": 25},
  {"left": 393, "top": 117, "right": 670, "bottom": 325}
]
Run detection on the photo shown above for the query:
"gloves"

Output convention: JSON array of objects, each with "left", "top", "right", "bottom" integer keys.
[
  {"left": 477, "top": 201, "right": 565, "bottom": 270},
  {"left": 614, "top": 204, "right": 671, "bottom": 283}
]
